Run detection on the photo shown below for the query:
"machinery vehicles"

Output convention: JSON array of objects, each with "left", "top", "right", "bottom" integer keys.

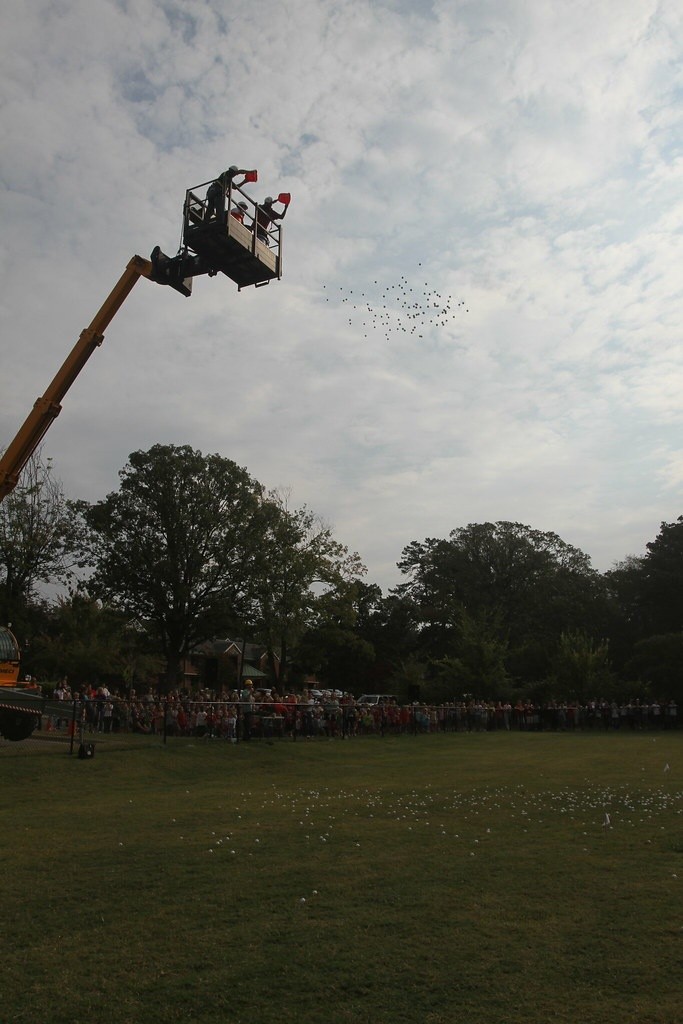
[{"left": 0, "top": 165, "right": 292, "bottom": 743}]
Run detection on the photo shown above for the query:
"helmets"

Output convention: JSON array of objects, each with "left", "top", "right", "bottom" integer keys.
[
  {"left": 229, "top": 165, "right": 239, "bottom": 171},
  {"left": 245, "top": 679, "right": 253, "bottom": 685},
  {"left": 237, "top": 201, "right": 249, "bottom": 209},
  {"left": 265, "top": 197, "right": 272, "bottom": 203}
]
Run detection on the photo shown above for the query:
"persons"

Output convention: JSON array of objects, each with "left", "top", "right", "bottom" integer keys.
[
  {"left": 231, "top": 201, "right": 248, "bottom": 224},
  {"left": 250, "top": 197, "right": 288, "bottom": 238},
  {"left": 378, "top": 697, "right": 683, "bottom": 735},
  {"left": 201, "top": 165, "right": 253, "bottom": 228},
  {"left": 25, "top": 678, "right": 378, "bottom": 744}
]
